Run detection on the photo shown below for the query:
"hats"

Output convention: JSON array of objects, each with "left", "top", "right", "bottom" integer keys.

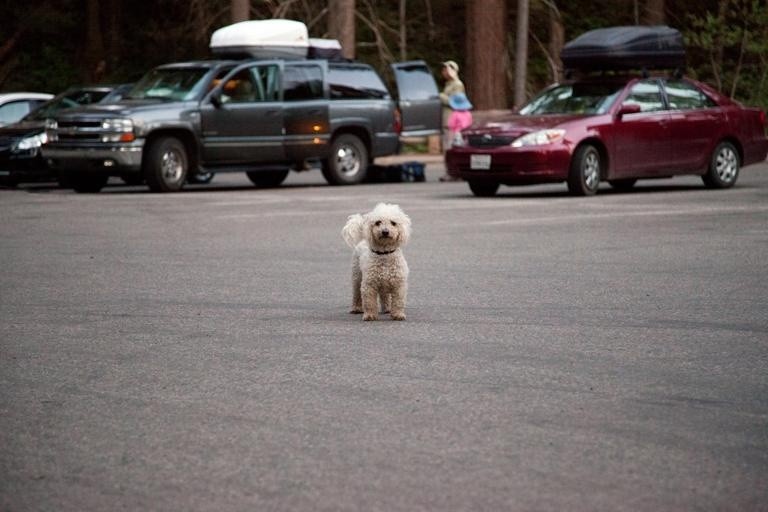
[
  {"left": 448, "top": 93, "right": 473, "bottom": 110},
  {"left": 441, "top": 58, "right": 460, "bottom": 79}
]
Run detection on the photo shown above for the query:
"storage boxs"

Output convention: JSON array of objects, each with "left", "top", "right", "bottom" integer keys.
[
  {"left": 388, "top": 163, "right": 424, "bottom": 183},
  {"left": 362, "top": 165, "right": 391, "bottom": 181}
]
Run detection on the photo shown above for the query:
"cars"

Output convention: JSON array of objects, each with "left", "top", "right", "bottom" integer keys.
[
  {"left": 0, "top": 93, "right": 79, "bottom": 131},
  {"left": 0, "top": 83, "right": 214, "bottom": 193},
  {"left": 443, "top": 23, "right": 765, "bottom": 199}
]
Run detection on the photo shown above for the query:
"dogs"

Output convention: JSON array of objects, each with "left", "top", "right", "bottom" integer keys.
[{"left": 341, "top": 203, "right": 413, "bottom": 321}]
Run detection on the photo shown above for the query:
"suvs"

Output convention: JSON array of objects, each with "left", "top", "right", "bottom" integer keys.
[{"left": 42, "top": 14, "right": 444, "bottom": 184}]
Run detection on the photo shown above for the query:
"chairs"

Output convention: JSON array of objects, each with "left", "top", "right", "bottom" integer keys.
[{"left": 232, "top": 82, "right": 255, "bottom": 102}]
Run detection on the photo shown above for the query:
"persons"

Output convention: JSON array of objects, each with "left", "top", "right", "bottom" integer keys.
[{"left": 438, "top": 60, "right": 475, "bottom": 181}]
